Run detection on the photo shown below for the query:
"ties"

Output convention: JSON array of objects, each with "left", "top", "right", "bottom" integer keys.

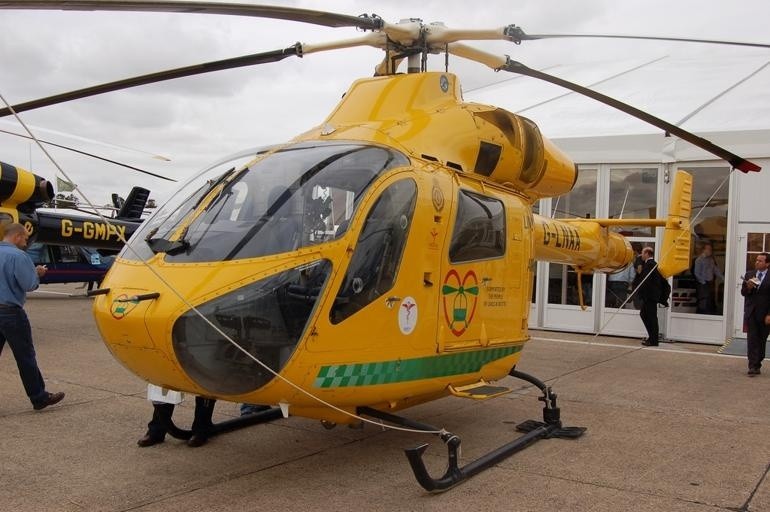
[{"left": 756, "top": 272, "right": 763, "bottom": 289}]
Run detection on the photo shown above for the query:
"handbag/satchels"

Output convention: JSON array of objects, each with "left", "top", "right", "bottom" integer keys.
[{"left": 655, "top": 269, "right": 670, "bottom": 307}]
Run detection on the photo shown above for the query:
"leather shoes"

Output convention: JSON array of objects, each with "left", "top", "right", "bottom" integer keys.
[
  {"left": 137, "top": 432, "right": 165, "bottom": 447},
  {"left": 747, "top": 368, "right": 762, "bottom": 376},
  {"left": 32, "top": 390, "right": 65, "bottom": 411},
  {"left": 187, "top": 435, "right": 210, "bottom": 447},
  {"left": 642, "top": 341, "right": 651, "bottom": 346}
]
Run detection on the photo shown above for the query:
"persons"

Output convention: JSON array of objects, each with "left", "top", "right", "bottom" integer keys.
[
  {"left": 694, "top": 244, "right": 724, "bottom": 314},
  {"left": 690, "top": 241, "right": 718, "bottom": 315},
  {"left": 607, "top": 263, "right": 637, "bottom": 309},
  {"left": 637, "top": 247, "right": 671, "bottom": 346},
  {"left": 741, "top": 253, "right": 770, "bottom": 376},
  {"left": 0, "top": 223, "right": 65, "bottom": 410},
  {"left": 136, "top": 395, "right": 217, "bottom": 448},
  {"left": 632, "top": 244, "right": 645, "bottom": 309}
]
